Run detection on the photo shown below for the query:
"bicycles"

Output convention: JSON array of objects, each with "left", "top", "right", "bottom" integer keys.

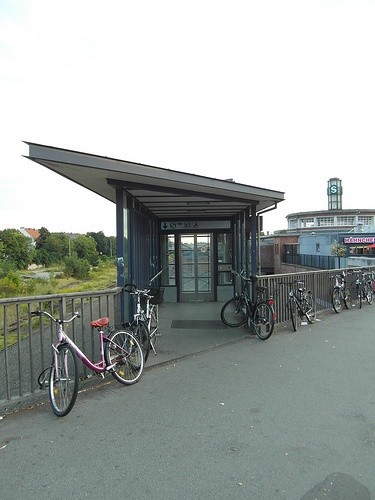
[
  {"left": 31, "top": 310, "right": 144, "bottom": 416},
  {"left": 329, "top": 270, "right": 351, "bottom": 313},
  {"left": 278, "top": 278, "right": 316, "bottom": 332},
  {"left": 347, "top": 268, "right": 374, "bottom": 309},
  {"left": 123, "top": 283, "right": 164, "bottom": 364},
  {"left": 220, "top": 268, "right": 276, "bottom": 341}
]
